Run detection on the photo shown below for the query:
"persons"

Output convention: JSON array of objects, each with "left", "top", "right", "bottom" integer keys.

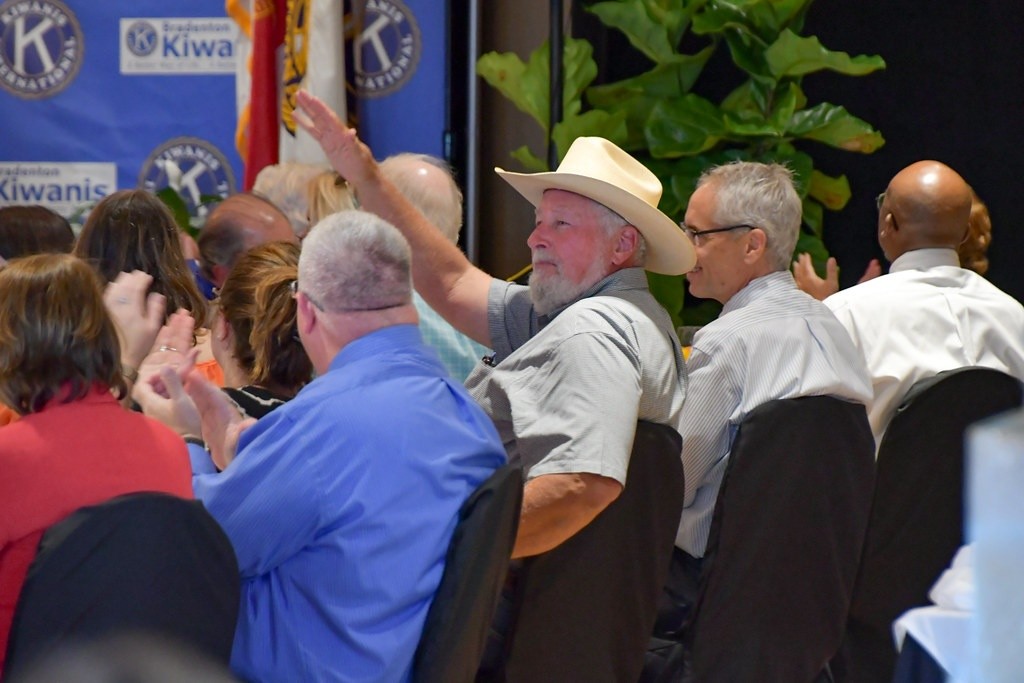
[
  {"left": 132, "top": 210, "right": 507, "bottom": 683},
  {"left": 653, "top": 163, "right": 875, "bottom": 639},
  {"left": 0, "top": 205, "right": 76, "bottom": 266},
  {"left": 179, "top": 195, "right": 299, "bottom": 304},
  {"left": 352, "top": 155, "right": 496, "bottom": 383},
  {"left": 0, "top": 255, "right": 193, "bottom": 664},
  {"left": 103, "top": 239, "right": 313, "bottom": 473},
  {"left": 793, "top": 160, "right": 1024, "bottom": 455},
  {"left": 71, "top": 189, "right": 208, "bottom": 349},
  {"left": 285, "top": 89, "right": 697, "bottom": 673},
  {"left": 253, "top": 164, "right": 359, "bottom": 242}
]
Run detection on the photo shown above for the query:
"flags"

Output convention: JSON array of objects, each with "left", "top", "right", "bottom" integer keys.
[{"left": 224, "top": 0, "right": 310, "bottom": 192}]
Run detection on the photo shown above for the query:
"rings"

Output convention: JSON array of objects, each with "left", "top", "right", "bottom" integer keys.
[
  {"left": 118, "top": 297, "right": 127, "bottom": 303},
  {"left": 159, "top": 347, "right": 176, "bottom": 351}
]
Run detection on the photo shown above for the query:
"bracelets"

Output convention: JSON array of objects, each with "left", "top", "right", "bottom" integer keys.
[{"left": 182, "top": 434, "right": 211, "bottom": 456}]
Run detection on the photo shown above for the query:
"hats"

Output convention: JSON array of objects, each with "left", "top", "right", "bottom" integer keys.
[{"left": 495, "top": 137, "right": 698, "bottom": 276}]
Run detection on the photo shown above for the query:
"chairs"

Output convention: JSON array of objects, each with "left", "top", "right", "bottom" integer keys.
[
  {"left": 2, "top": 491, "right": 241, "bottom": 683},
  {"left": 412, "top": 462, "right": 524, "bottom": 683},
  {"left": 652, "top": 393, "right": 876, "bottom": 683},
  {"left": 830, "top": 366, "right": 1024, "bottom": 683},
  {"left": 492, "top": 420, "right": 685, "bottom": 683}
]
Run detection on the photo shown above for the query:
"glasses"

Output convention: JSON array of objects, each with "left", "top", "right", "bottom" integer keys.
[
  {"left": 876, "top": 191, "right": 901, "bottom": 234},
  {"left": 335, "top": 175, "right": 357, "bottom": 204},
  {"left": 678, "top": 222, "right": 755, "bottom": 243},
  {"left": 290, "top": 281, "right": 324, "bottom": 312}
]
